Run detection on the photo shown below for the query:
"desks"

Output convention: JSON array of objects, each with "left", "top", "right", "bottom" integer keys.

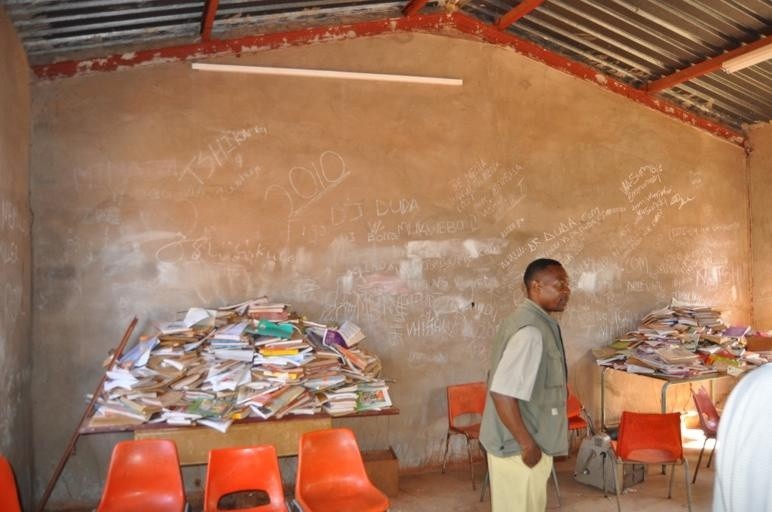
[
  {"left": 79, "top": 405, "right": 401, "bottom": 467},
  {"left": 595, "top": 361, "right": 728, "bottom": 427}
]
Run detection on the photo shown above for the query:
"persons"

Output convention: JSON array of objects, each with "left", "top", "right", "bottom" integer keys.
[{"left": 477, "top": 258, "right": 571, "bottom": 512}]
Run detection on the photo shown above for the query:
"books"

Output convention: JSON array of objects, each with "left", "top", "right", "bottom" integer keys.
[
  {"left": 590, "top": 299, "right": 769, "bottom": 380},
  {"left": 83, "top": 292, "right": 396, "bottom": 434}
]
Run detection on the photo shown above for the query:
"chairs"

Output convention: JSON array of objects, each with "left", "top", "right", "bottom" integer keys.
[
  {"left": 602, "top": 410, "right": 693, "bottom": 512},
  {"left": 479, "top": 385, "right": 596, "bottom": 506},
  {"left": 693, "top": 385, "right": 720, "bottom": 484},
  {"left": 292, "top": 428, "right": 391, "bottom": 510},
  {"left": 205, "top": 446, "right": 290, "bottom": 512},
  {"left": 0, "top": 455, "right": 22, "bottom": 512},
  {"left": 97, "top": 439, "right": 192, "bottom": 512},
  {"left": 441, "top": 382, "right": 488, "bottom": 490}
]
